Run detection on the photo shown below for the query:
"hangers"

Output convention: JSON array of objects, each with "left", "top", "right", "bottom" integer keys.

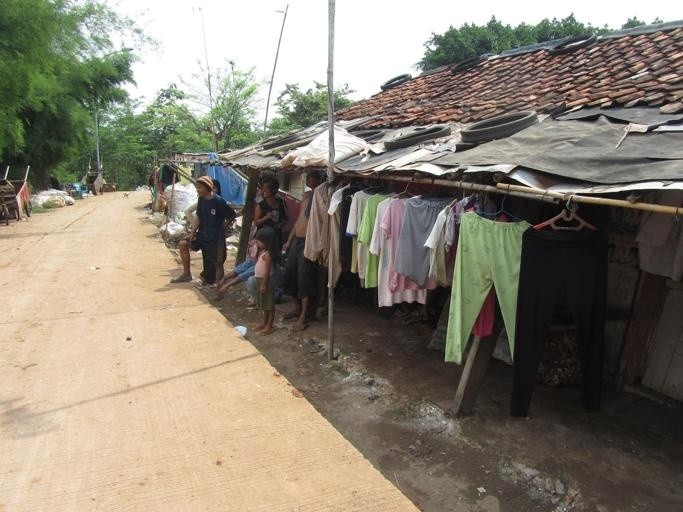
[
  {"left": 472, "top": 188, "right": 523, "bottom": 222},
  {"left": 361, "top": 172, "right": 466, "bottom": 203},
  {"left": 529, "top": 191, "right": 600, "bottom": 234}
]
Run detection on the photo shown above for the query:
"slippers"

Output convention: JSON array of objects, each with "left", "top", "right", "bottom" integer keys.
[{"left": 252, "top": 324, "right": 277, "bottom": 336}]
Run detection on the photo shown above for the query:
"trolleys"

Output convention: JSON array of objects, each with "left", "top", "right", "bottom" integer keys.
[
  {"left": 80, "top": 155, "right": 103, "bottom": 195},
  {"left": 0, "top": 165, "right": 32, "bottom": 220}
]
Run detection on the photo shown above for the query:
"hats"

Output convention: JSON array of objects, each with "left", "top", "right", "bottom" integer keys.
[{"left": 196, "top": 175, "right": 214, "bottom": 191}]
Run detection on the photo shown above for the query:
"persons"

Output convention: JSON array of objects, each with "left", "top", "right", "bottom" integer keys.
[
  {"left": 251, "top": 225, "right": 279, "bottom": 336},
  {"left": 248, "top": 175, "right": 282, "bottom": 240},
  {"left": 253, "top": 174, "right": 289, "bottom": 261},
  {"left": 282, "top": 171, "right": 323, "bottom": 332},
  {"left": 215, "top": 237, "right": 285, "bottom": 311},
  {"left": 168, "top": 174, "right": 238, "bottom": 288}
]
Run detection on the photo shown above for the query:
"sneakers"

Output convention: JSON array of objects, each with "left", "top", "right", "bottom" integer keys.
[{"left": 171, "top": 272, "right": 192, "bottom": 283}]
